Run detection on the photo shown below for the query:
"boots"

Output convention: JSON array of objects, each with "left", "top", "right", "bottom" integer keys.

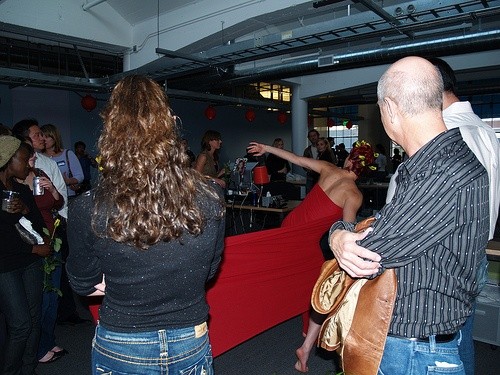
[{"left": 56, "top": 293, "right": 75, "bottom": 326}]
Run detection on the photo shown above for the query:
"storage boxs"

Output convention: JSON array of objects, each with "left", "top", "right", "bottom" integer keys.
[{"left": 472, "top": 282, "right": 500, "bottom": 347}]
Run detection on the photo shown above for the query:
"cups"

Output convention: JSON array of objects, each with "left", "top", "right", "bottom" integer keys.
[{"left": 2, "top": 190, "right": 20, "bottom": 212}]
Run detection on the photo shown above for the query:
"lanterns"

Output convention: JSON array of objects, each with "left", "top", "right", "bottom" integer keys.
[
  {"left": 204, "top": 107, "right": 216, "bottom": 120},
  {"left": 346, "top": 122, "right": 352, "bottom": 129},
  {"left": 278, "top": 113, "right": 287, "bottom": 123},
  {"left": 327, "top": 119, "right": 335, "bottom": 127},
  {"left": 246, "top": 111, "right": 255, "bottom": 121},
  {"left": 81, "top": 95, "right": 97, "bottom": 112}
]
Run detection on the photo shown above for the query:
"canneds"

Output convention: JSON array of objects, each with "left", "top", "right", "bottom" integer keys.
[
  {"left": 2, "top": 190, "right": 15, "bottom": 211},
  {"left": 33, "top": 177, "right": 45, "bottom": 195}
]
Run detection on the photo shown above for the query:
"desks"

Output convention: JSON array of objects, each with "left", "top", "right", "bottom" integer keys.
[
  {"left": 286, "top": 180, "right": 389, "bottom": 217},
  {"left": 226, "top": 198, "right": 303, "bottom": 227}
]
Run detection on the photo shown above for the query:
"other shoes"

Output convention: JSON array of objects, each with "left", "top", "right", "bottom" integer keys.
[
  {"left": 39, "top": 352, "right": 64, "bottom": 364},
  {"left": 53, "top": 349, "right": 68, "bottom": 355}
]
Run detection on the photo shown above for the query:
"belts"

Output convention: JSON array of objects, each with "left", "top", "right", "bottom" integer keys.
[{"left": 393, "top": 332, "right": 458, "bottom": 343}]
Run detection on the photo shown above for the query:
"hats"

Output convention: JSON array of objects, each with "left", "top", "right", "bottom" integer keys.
[{"left": 0, "top": 135, "right": 21, "bottom": 169}]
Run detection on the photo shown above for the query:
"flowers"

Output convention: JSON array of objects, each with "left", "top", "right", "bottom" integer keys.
[{"left": 40, "top": 218, "right": 63, "bottom": 298}]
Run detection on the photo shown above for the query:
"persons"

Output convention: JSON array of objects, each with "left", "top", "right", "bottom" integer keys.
[
  {"left": 327, "top": 55, "right": 490, "bottom": 375},
  {"left": 328, "top": 137, "right": 350, "bottom": 167},
  {"left": 181, "top": 138, "right": 196, "bottom": 168},
  {"left": 302, "top": 129, "right": 320, "bottom": 195},
  {"left": 0, "top": 118, "right": 99, "bottom": 375},
  {"left": 374, "top": 143, "right": 387, "bottom": 172},
  {"left": 266, "top": 137, "right": 292, "bottom": 227},
  {"left": 244, "top": 148, "right": 257, "bottom": 163},
  {"left": 194, "top": 130, "right": 227, "bottom": 190},
  {"left": 392, "top": 149, "right": 401, "bottom": 174},
  {"left": 386, "top": 55, "right": 500, "bottom": 375},
  {"left": 64, "top": 74, "right": 226, "bottom": 375},
  {"left": 90, "top": 139, "right": 380, "bottom": 372},
  {"left": 308, "top": 137, "right": 336, "bottom": 185}
]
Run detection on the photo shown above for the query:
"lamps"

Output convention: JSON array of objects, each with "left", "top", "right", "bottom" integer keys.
[{"left": 395, "top": 4, "right": 416, "bottom": 16}]
